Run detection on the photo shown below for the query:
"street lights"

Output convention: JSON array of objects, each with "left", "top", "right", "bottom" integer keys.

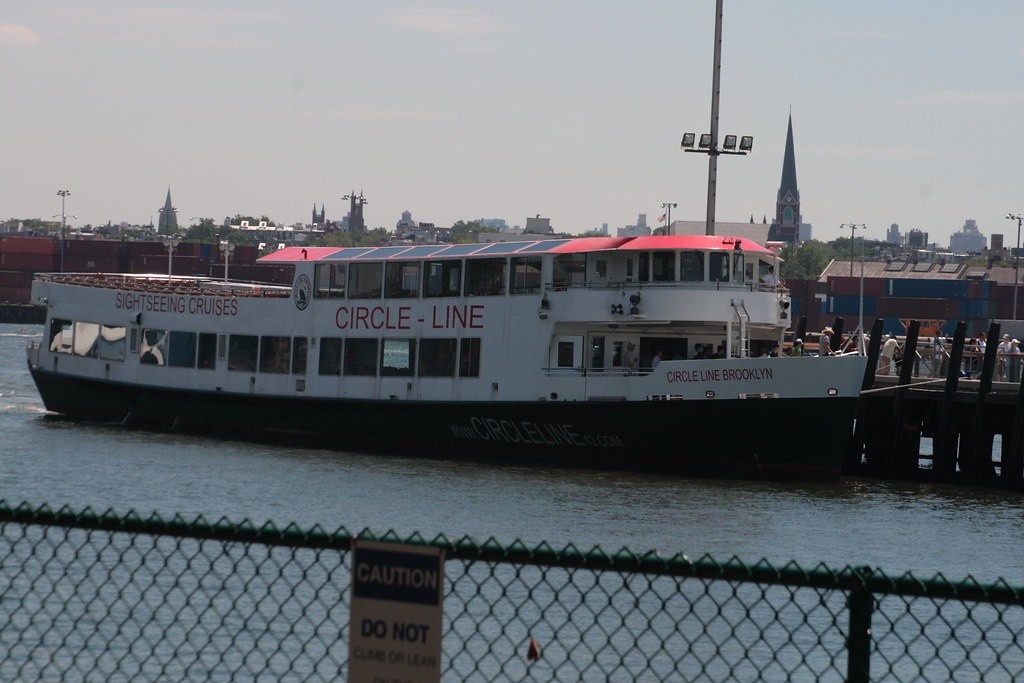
[
  {"left": 661, "top": 202, "right": 678, "bottom": 236},
  {"left": 57, "top": 189, "right": 71, "bottom": 217},
  {"left": 1003, "top": 213, "right": 1024, "bottom": 321},
  {"left": 837, "top": 222, "right": 869, "bottom": 280},
  {"left": 682, "top": 131, "right": 752, "bottom": 233}
]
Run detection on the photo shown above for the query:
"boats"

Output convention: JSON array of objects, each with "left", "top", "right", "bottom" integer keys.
[{"left": 20, "top": 236, "right": 870, "bottom": 471}]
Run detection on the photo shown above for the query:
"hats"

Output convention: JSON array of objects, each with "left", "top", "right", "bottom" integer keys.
[
  {"left": 796, "top": 339, "right": 803, "bottom": 344},
  {"left": 1003, "top": 333, "right": 1010, "bottom": 339},
  {"left": 822, "top": 326, "right": 834, "bottom": 334},
  {"left": 1011, "top": 339, "right": 1020, "bottom": 344}
]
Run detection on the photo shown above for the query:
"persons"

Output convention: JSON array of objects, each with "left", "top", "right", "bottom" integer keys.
[
  {"left": 791, "top": 339, "right": 804, "bottom": 356},
  {"left": 1005, "top": 339, "right": 1024, "bottom": 383},
  {"left": 689, "top": 343, "right": 724, "bottom": 360},
  {"left": 651, "top": 349, "right": 663, "bottom": 367},
  {"left": 763, "top": 266, "right": 784, "bottom": 288},
  {"left": 878, "top": 336, "right": 903, "bottom": 374},
  {"left": 996, "top": 334, "right": 1011, "bottom": 382},
  {"left": 976, "top": 332, "right": 987, "bottom": 353},
  {"left": 925, "top": 331, "right": 953, "bottom": 378},
  {"left": 623, "top": 343, "right": 636, "bottom": 376},
  {"left": 770, "top": 345, "right": 780, "bottom": 357},
  {"left": 818, "top": 326, "right": 837, "bottom": 356},
  {"left": 964, "top": 338, "right": 981, "bottom": 379},
  {"left": 840, "top": 334, "right": 870, "bottom": 356}
]
